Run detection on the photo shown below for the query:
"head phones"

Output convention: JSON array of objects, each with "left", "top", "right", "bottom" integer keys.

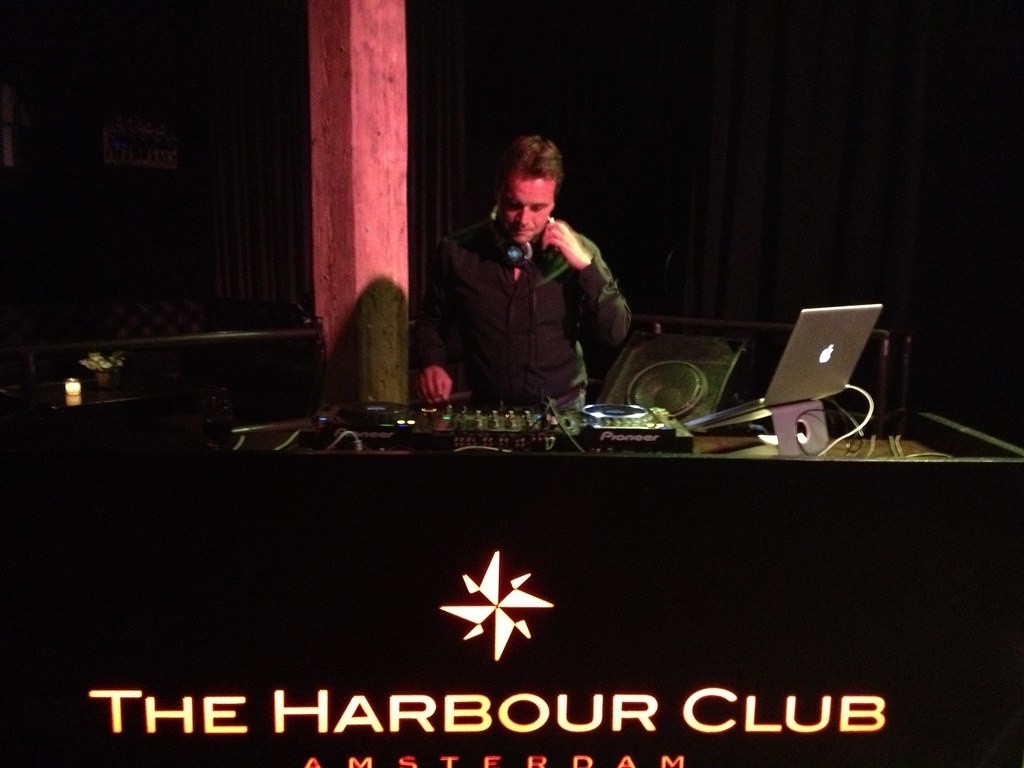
[{"left": 489, "top": 206, "right": 559, "bottom": 267}]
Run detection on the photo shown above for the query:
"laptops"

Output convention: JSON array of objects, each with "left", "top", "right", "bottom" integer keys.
[{"left": 685, "top": 303, "right": 883, "bottom": 428}]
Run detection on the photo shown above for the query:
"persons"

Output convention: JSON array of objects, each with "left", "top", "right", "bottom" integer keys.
[{"left": 406, "top": 136, "right": 631, "bottom": 413}]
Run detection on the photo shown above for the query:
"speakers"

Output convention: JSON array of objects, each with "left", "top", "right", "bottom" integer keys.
[{"left": 593, "top": 329, "right": 774, "bottom": 422}]
